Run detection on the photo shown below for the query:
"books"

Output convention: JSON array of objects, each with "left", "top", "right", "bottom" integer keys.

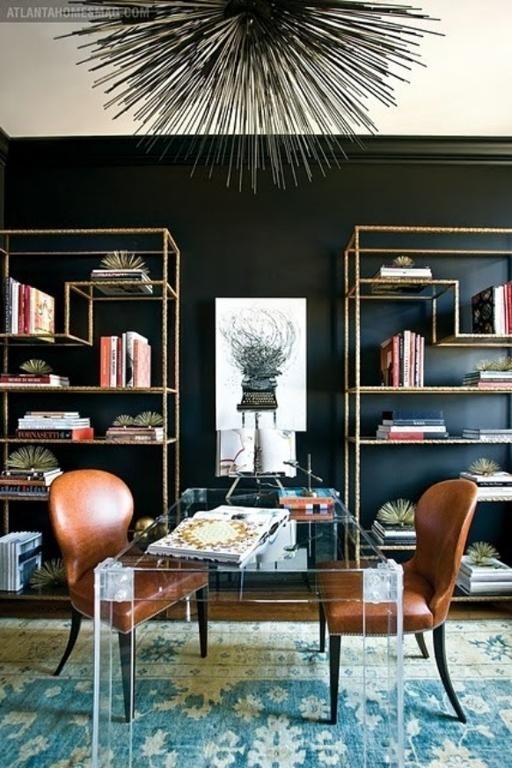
[
  {"left": 459, "top": 471, "right": 512, "bottom": 497},
  {"left": 471, "top": 280, "right": 512, "bottom": 335},
  {"left": 17, "top": 411, "right": 94, "bottom": 441},
  {"left": 99, "top": 331, "right": 153, "bottom": 388},
  {"left": 0, "top": 373, "right": 70, "bottom": 387},
  {"left": 374, "top": 267, "right": 433, "bottom": 294},
  {"left": 455, "top": 556, "right": 512, "bottom": 596},
  {"left": 372, "top": 519, "right": 417, "bottom": 545},
  {"left": 379, "top": 330, "right": 425, "bottom": 387},
  {"left": 375, "top": 410, "right": 512, "bottom": 439},
  {"left": 91, "top": 270, "right": 154, "bottom": 296},
  {"left": 105, "top": 426, "right": 165, "bottom": 441},
  {"left": 0, "top": 531, "right": 43, "bottom": 591},
  {"left": 1, "top": 277, "right": 56, "bottom": 344},
  {"left": 216, "top": 427, "right": 292, "bottom": 476},
  {"left": 463, "top": 370, "right": 512, "bottom": 388},
  {"left": 145, "top": 505, "right": 290, "bottom": 564},
  {"left": 1, "top": 467, "right": 64, "bottom": 500}
]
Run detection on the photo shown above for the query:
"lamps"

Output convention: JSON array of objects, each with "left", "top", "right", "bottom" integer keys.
[{"left": 51, "top": 0, "right": 445, "bottom": 198}]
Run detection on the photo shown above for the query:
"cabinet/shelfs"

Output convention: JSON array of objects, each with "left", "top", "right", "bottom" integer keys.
[
  {"left": 1, "top": 226, "right": 180, "bottom": 535},
  {"left": 343, "top": 224, "right": 511, "bottom": 604}
]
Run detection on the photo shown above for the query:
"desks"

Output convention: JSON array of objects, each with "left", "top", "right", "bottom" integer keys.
[{"left": 93, "top": 486, "right": 405, "bottom": 768}]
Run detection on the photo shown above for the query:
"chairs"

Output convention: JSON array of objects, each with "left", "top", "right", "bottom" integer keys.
[
  {"left": 48, "top": 468, "right": 210, "bottom": 724},
  {"left": 314, "top": 477, "right": 480, "bottom": 725}
]
